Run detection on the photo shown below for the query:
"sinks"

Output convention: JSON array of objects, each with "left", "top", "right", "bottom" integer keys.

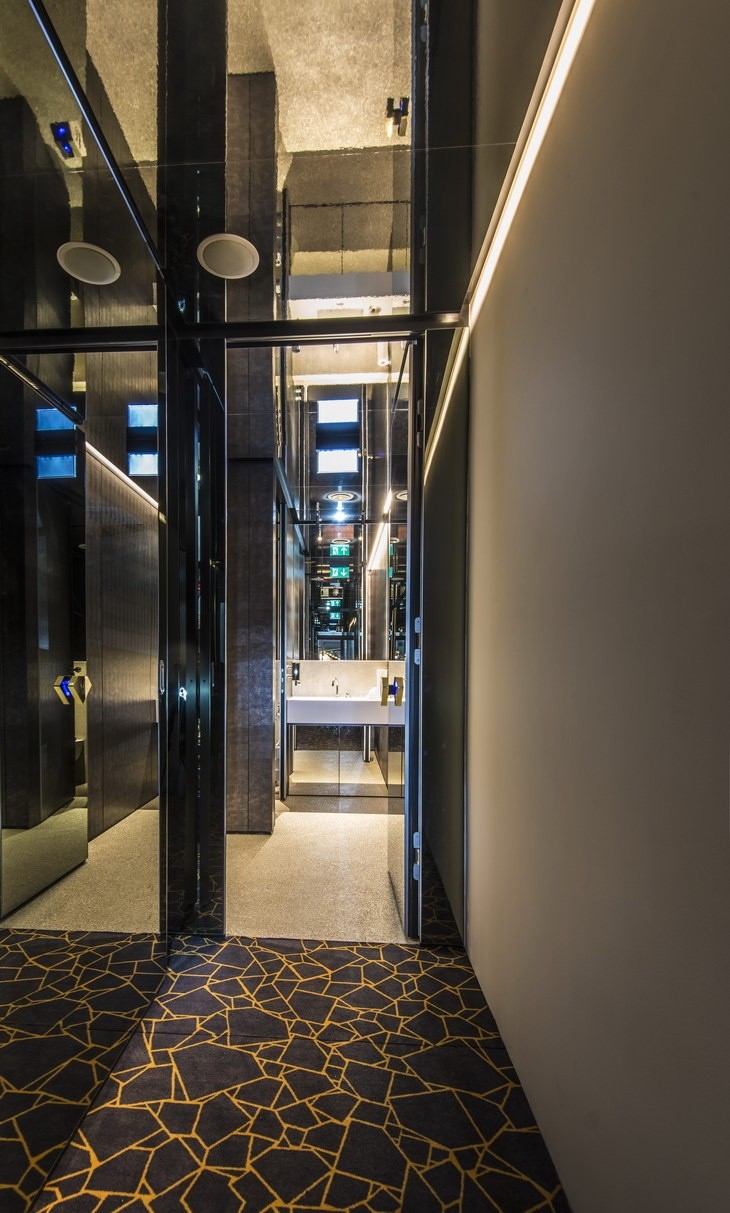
[
  {"left": 389, "top": 698, "right": 406, "bottom": 724},
  {"left": 285, "top": 696, "right": 389, "bottom": 725}
]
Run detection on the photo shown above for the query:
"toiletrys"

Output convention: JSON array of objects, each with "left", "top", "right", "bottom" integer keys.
[{"left": 291, "top": 663, "right": 301, "bottom": 687}]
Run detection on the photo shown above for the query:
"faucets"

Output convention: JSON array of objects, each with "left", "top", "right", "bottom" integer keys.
[{"left": 331, "top": 677, "right": 339, "bottom": 697}]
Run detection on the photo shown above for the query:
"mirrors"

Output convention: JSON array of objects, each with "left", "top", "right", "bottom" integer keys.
[
  {"left": 0, "top": 1, "right": 174, "bottom": 1213},
  {"left": 293, "top": 521, "right": 407, "bottom": 660}
]
